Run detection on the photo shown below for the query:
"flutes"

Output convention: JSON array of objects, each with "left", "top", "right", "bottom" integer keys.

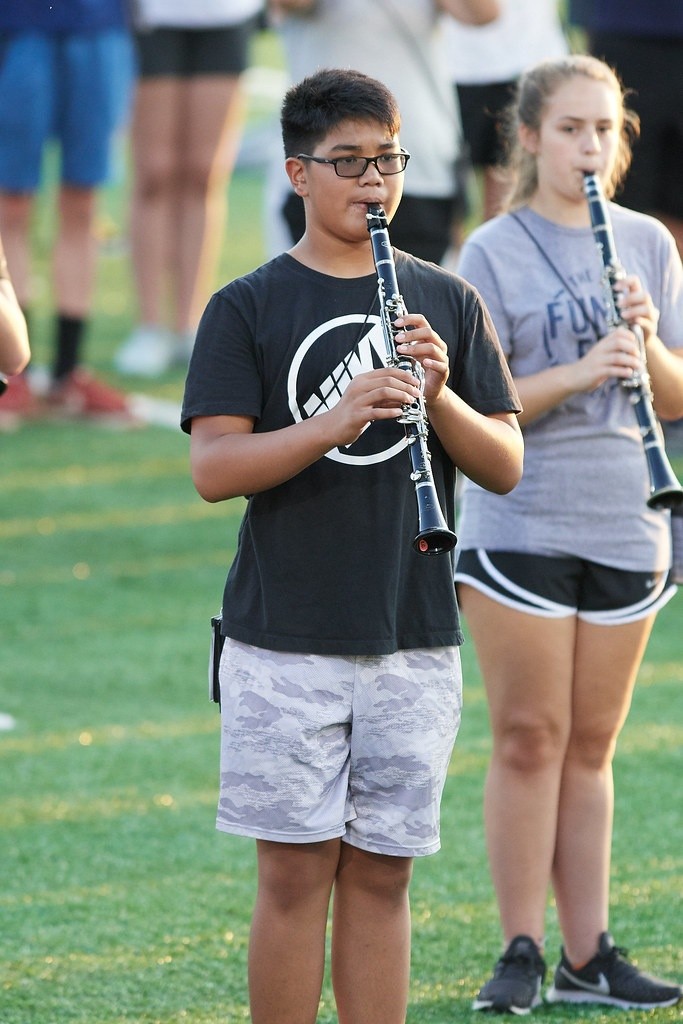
[
  {"left": 583, "top": 171, "right": 683, "bottom": 511},
  {"left": 367, "top": 201, "right": 458, "bottom": 557}
]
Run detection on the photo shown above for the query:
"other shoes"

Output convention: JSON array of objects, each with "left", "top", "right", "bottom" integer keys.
[
  {"left": 47, "top": 368, "right": 146, "bottom": 421},
  {"left": 0, "top": 372, "right": 41, "bottom": 420}
]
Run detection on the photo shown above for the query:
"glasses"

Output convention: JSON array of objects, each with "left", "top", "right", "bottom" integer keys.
[{"left": 296, "top": 148, "right": 410, "bottom": 178}]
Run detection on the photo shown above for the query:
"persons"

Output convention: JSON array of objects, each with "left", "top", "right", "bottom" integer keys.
[
  {"left": 457, "top": 56, "right": 683, "bottom": 1016},
  {"left": 0, "top": 0, "right": 683, "bottom": 436},
  {"left": 181, "top": 68, "right": 525, "bottom": 1024}
]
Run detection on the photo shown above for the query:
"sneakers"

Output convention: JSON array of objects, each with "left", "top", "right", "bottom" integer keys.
[
  {"left": 471, "top": 936, "right": 546, "bottom": 1015},
  {"left": 546, "top": 931, "right": 683, "bottom": 1008}
]
